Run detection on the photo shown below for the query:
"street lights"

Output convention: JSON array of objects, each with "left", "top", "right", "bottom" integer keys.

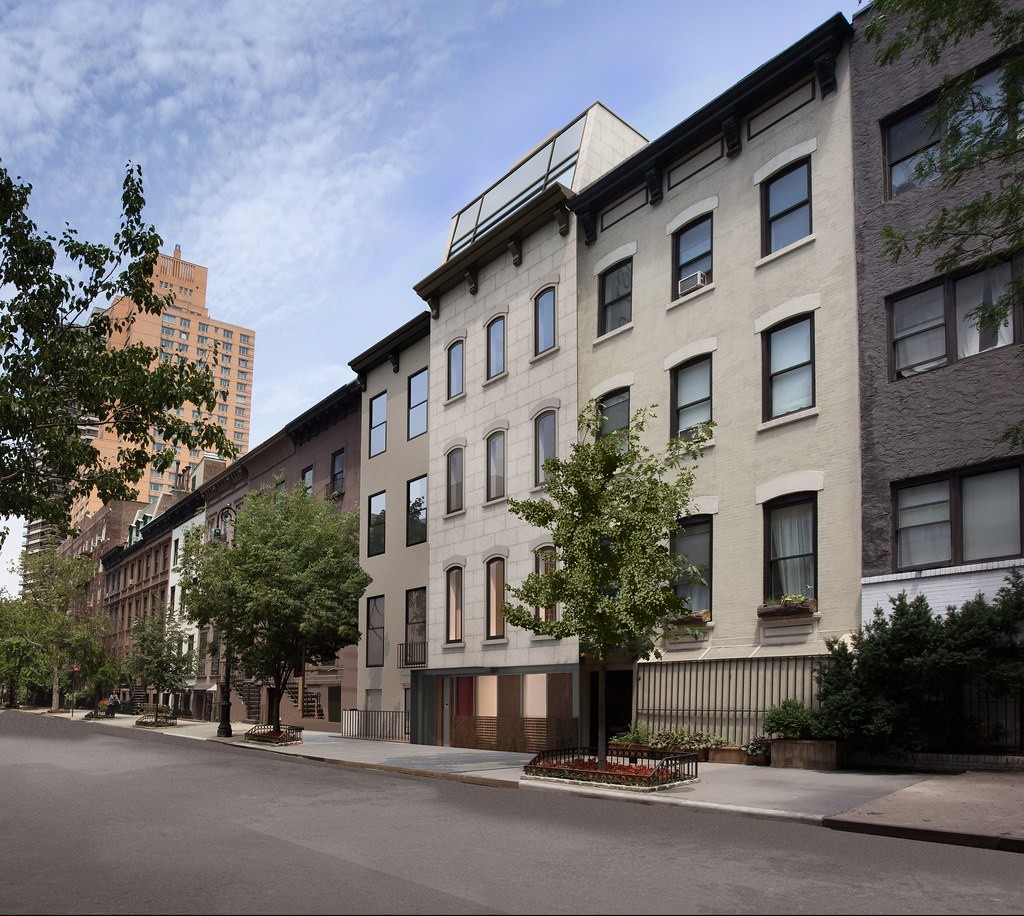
[{"left": 213, "top": 506, "right": 237, "bottom": 738}]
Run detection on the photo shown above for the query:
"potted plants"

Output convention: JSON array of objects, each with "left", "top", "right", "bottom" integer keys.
[
  {"left": 761, "top": 697, "right": 841, "bottom": 769},
  {"left": 705, "top": 726, "right": 748, "bottom": 764},
  {"left": 739, "top": 730, "right": 771, "bottom": 766},
  {"left": 668, "top": 609, "right": 711, "bottom": 627},
  {"left": 757, "top": 584, "right": 817, "bottom": 617},
  {"left": 648, "top": 723, "right": 708, "bottom": 761},
  {"left": 608, "top": 719, "right": 655, "bottom": 759}
]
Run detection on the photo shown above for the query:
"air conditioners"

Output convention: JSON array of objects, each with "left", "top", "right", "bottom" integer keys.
[
  {"left": 678, "top": 271, "right": 707, "bottom": 297},
  {"left": 678, "top": 423, "right": 707, "bottom": 447}
]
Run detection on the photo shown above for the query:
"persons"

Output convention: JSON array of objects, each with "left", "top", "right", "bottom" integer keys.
[{"left": 108, "top": 690, "right": 121, "bottom": 718}]
